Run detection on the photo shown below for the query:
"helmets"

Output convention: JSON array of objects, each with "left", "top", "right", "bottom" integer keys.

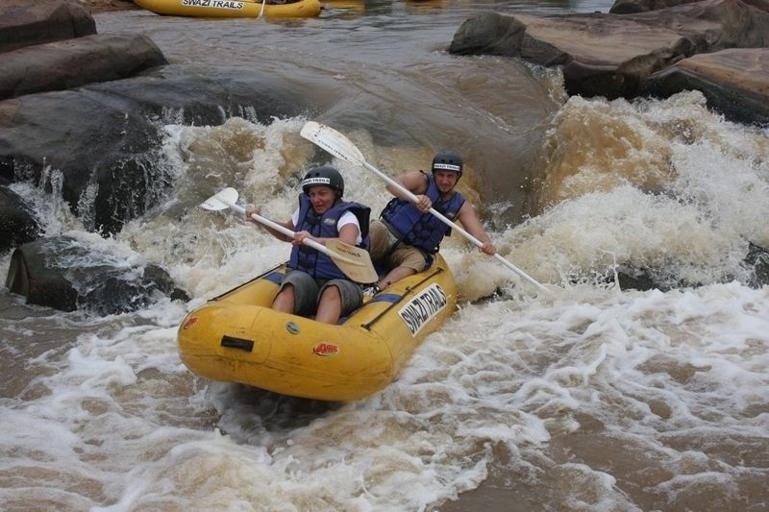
[
  {"left": 431, "top": 151, "right": 464, "bottom": 173},
  {"left": 301, "top": 167, "right": 345, "bottom": 191}
]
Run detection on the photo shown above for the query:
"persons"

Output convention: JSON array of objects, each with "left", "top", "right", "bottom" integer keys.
[
  {"left": 246, "top": 167, "right": 365, "bottom": 326},
  {"left": 364, "top": 150, "right": 496, "bottom": 304}
]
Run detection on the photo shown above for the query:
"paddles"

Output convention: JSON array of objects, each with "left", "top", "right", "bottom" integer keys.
[
  {"left": 300, "top": 120, "right": 547, "bottom": 294},
  {"left": 200, "top": 186, "right": 379, "bottom": 286}
]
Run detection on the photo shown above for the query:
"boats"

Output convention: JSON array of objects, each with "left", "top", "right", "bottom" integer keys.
[
  {"left": 134, "top": 0, "right": 322, "bottom": 19},
  {"left": 178, "top": 251, "right": 457, "bottom": 401}
]
